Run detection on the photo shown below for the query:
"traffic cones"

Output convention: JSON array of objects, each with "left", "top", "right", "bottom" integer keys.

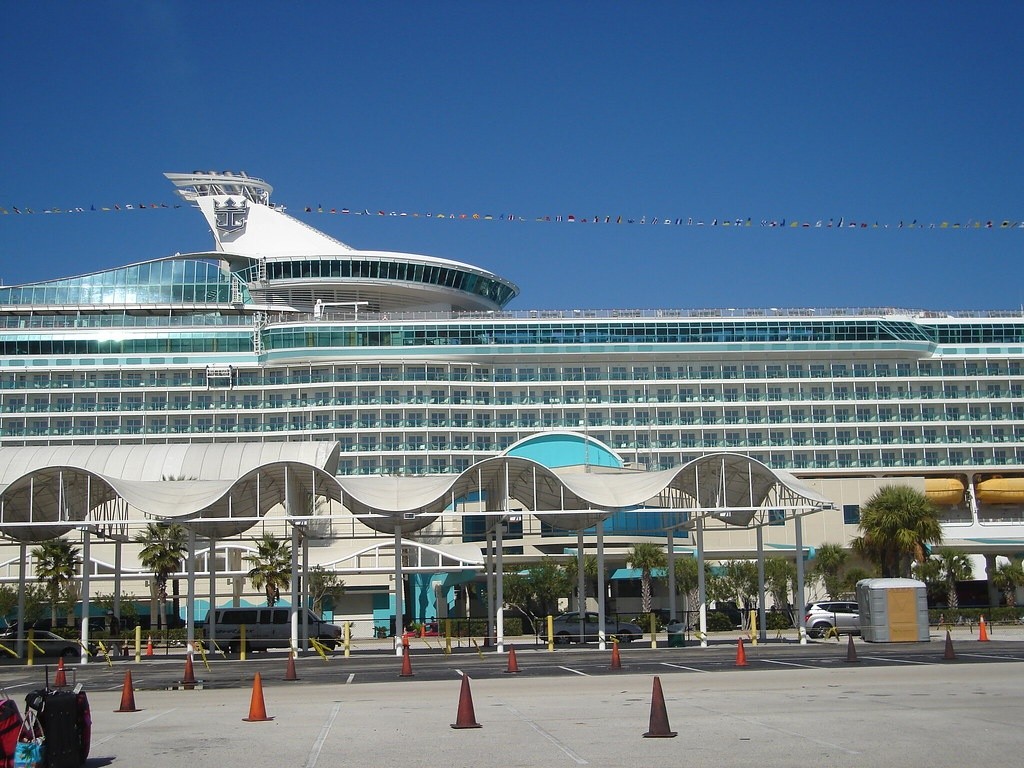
[
  {"left": 399, "top": 647, "right": 414, "bottom": 677},
  {"left": 449, "top": 673, "right": 483, "bottom": 729},
  {"left": 607, "top": 640, "right": 625, "bottom": 670},
  {"left": 51, "top": 657, "right": 70, "bottom": 687},
  {"left": 939, "top": 612, "right": 992, "bottom": 661},
  {"left": 124, "top": 639, "right": 129, "bottom": 656},
  {"left": 241, "top": 671, "right": 275, "bottom": 721},
  {"left": 642, "top": 675, "right": 678, "bottom": 738},
  {"left": 113, "top": 671, "right": 142, "bottom": 712},
  {"left": 503, "top": 644, "right": 522, "bottom": 673},
  {"left": 843, "top": 634, "right": 862, "bottom": 662},
  {"left": 144, "top": 635, "right": 154, "bottom": 655},
  {"left": 281, "top": 652, "right": 301, "bottom": 681},
  {"left": 734, "top": 637, "right": 749, "bottom": 667},
  {"left": 181, "top": 652, "right": 198, "bottom": 684}
]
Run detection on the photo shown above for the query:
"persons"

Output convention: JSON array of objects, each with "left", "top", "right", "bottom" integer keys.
[
  {"left": 430, "top": 616, "right": 435, "bottom": 631},
  {"left": 104, "top": 610, "right": 120, "bottom": 655},
  {"left": 938, "top": 613, "right": 964, "bottom": 625}
]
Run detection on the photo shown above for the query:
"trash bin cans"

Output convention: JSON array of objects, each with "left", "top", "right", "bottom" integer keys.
[{"left": 667, "top": 619, "right": 687, "bottom": 647}]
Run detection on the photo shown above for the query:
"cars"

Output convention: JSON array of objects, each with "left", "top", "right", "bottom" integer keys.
[
  {"left": 0, "top": 630, "right": 99, "bottom": 658},
  {"left": 404, "top": 622, "right": 464, "bottom": 637},
  {"left": 538, "top": 610, "right": 643, "bottom": 644},
  {"left": 804, "top": 600, "right": 862, "bottom": 639}
]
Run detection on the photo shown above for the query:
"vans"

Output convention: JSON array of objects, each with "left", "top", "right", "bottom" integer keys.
[{"left": 202, "top": 606, "right": 342, "bottom": 654}]
